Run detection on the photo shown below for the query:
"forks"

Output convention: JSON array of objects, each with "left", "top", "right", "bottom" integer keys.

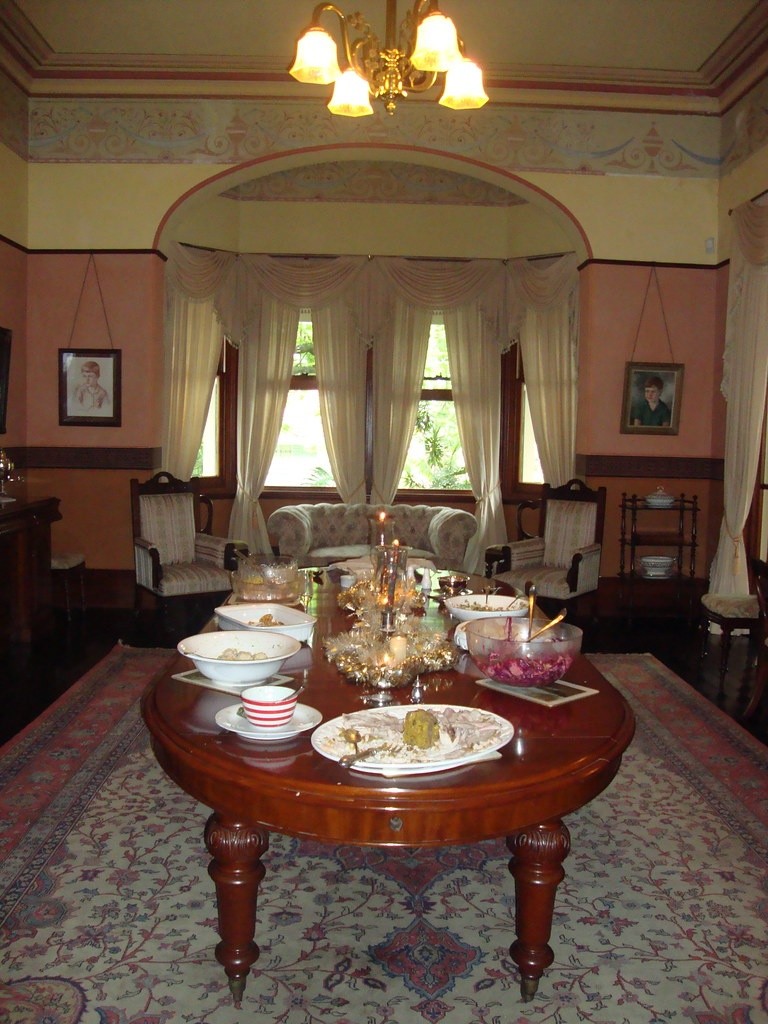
[{"left": 338, "top": 743, "right": 403, "bottom": 769}]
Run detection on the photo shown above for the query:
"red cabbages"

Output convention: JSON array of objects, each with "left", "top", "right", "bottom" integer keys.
[{"left": 472, "top": 616, "right": 573, "bottom": 688}]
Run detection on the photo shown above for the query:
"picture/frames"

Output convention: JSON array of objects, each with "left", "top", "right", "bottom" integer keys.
[
  {"left": 618, "top": 361, "right": 686, "bottom": 436},
  {"left": 58, "top": 348, "right": 124, "bottom": 428}
]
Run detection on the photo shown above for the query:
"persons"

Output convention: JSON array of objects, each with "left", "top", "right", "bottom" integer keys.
[
  {"left": 633, "top": 376, "right": 670, "bottom": 427},
  {"left": 71, "top": 360, "right": 108, "bottom": 411}
]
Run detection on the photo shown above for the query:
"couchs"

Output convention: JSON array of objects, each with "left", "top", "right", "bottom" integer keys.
[{"left": 266, "top": 503, "right": 480, "bottom": 575}]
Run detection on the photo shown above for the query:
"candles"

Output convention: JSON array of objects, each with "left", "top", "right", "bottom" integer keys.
[
  {"left": 389, "top": 635, "right": 406, "bottom": 660},
  {"left": 378, "top": 509, "right": 386, "bottom": 545},
  {"left": 391, "top": 539, "right": 400, "bottom": 564}
]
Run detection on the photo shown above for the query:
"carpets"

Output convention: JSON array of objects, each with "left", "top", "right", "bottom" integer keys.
[{"left": 0, "top": 639, "right": 768, "bottom": 1024}]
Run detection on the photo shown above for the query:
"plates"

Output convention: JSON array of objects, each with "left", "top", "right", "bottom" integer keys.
[
  {"left": 310, "top": 702, "right": 515, "bottom": 771},
  {"left": 214, "top": 703, "right": 324, "bottom": 741}
]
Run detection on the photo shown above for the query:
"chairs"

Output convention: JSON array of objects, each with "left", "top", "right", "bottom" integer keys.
[
  {"left": 485, "top": 477, "right": 607, "bottom": 618},
  {"left": 742, "top": 554, "right": 768, "bottom": 728},
  {"left": 130, "top": 470, "right": 238, "bottom": 630}
]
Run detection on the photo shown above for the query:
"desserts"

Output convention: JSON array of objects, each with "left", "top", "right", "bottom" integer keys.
[{"left": 403, "top": 708, "right": 440, "bottom": 750}]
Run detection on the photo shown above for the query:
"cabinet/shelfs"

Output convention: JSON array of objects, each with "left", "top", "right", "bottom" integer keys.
[{"left": 619, "top": 492, "right": 700, "bottom": 632}]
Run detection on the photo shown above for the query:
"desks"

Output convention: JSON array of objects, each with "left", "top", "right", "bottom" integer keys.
[{"left": 0, "top": 495, "right": 64, "bottom": 661}]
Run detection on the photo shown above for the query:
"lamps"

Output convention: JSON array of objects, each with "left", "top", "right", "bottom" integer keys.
[{"left": 289, "top": 0, "right": 489, "bottom": 118}]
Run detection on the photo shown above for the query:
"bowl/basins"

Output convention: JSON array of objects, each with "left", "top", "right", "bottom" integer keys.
[
  {"left": 213, "top": 602, "right": 318, "bottom": 642},
  {"left": 230, "top": 554, "right": 305, "bottom": 604},
  {"left": 641, "top": 556, "right": 673, "bottom": 576},
  {"left": 644, "top": 485, "right": 676, "bottom": 505},
  {"left": 464, "top": 616, "right": 583, "bottom": 687},
  {"left": 444, "top": 594, "right": 530, "bottom": 622},
  {"left": 177, "top": 630, "right": 302, "bottom": 687},
  {"left": 240, "top": 686, "right": 298, "bottom": 730}
]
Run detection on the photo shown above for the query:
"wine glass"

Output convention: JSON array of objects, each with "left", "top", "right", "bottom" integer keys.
[{"left": 0, "top": 450, "right": 14, "bottom": 495}]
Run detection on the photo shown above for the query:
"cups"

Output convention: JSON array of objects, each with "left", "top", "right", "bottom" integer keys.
[{"left": 438, "top": 575, "right": 471, "bottom": 597}]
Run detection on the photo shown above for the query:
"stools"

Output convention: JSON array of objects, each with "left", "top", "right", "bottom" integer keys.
[
  {"left": 701, "top": 593, "right": 760, "bottom": 674},
  {"left": 48, "top": 553, "right": 86, "bottom": 626}
]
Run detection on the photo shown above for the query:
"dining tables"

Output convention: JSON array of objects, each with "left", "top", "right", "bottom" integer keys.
[{"left": 140, "top": 571, "right": 637, "bottom": 1002}]
